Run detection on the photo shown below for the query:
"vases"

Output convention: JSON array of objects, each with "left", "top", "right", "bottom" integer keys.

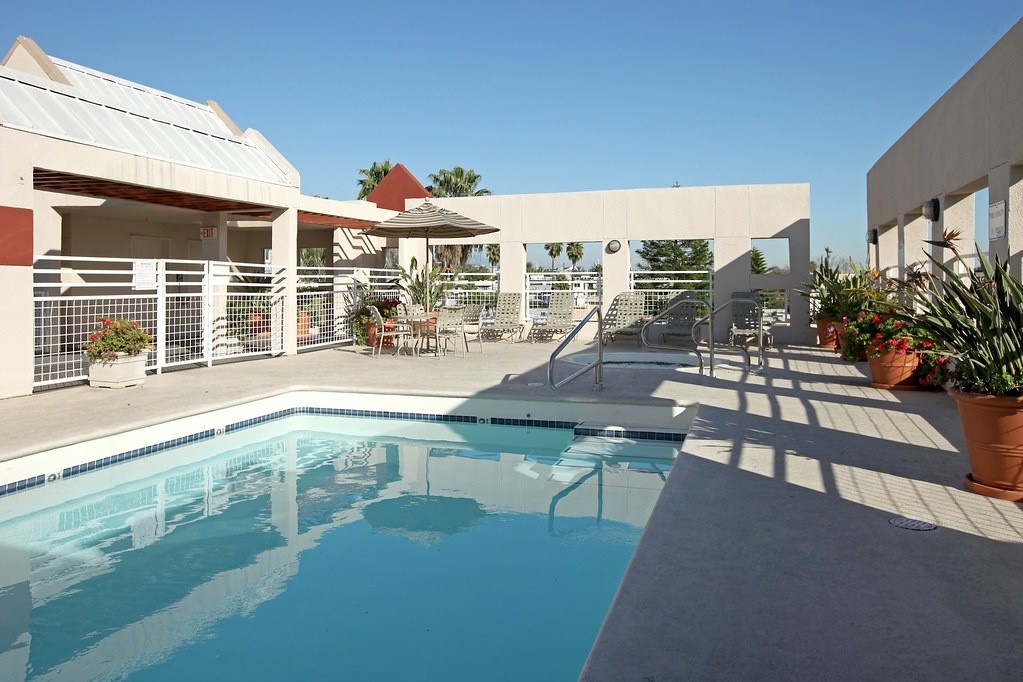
[{"left": 83, "top": 350, "right": 151, "bottom": 390}]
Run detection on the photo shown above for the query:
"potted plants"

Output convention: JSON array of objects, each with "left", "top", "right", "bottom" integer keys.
[
  {"left": 396, "top": 255, "right": 460, "bottom": 338},
  {"left": 219, "top": 266, "right": 329, "bottom": 346},
  {"left": 793, "top": 228, "right": 1023, "bottom": 503}
]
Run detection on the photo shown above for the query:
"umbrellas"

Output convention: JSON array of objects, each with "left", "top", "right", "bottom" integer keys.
[{"left": 357, "top": 196, "right": 500, "bottom": 350}]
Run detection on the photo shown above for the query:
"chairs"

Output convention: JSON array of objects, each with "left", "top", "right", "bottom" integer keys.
[
  {"left": 479, "top": 292, "right": 526, "bottom": 344},
  {"left": 415, "top": 305, "right": 466, "bottom": 358},
  {"left": 532, "top": 291, "right": 579, "bottom": 343},
  {"left": 602, "top": 290, "right": 649, "bottom": 349},
  {"left": 458, "top": 303, "right": 486, "bottom": 353},
  {"left": 396, "top": 303, "right": 435, "bottom": 358},
  {"left": 657, "top": 290, "right": 702, "bottom": 349},
  {"left": 365, "top": 305, "right": 415, "bottom": 358},
  {"left": 729, "top": 291, "right": 774, "bottom": 350}
]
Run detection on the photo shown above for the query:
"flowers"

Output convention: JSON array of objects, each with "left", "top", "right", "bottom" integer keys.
[
  {"left": 364, "top": 316, "right": 398, "bottom": 349},
  {"left": 356, "top": 297, "right": 402, "bottom": 319},
  {"left": 82, "top": 318, "right": 156, "bottom": 367}
]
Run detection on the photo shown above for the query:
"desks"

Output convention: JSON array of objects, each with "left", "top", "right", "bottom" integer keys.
[{"left": 390, "top": 314, "right": 439, "bottom": 359}]
